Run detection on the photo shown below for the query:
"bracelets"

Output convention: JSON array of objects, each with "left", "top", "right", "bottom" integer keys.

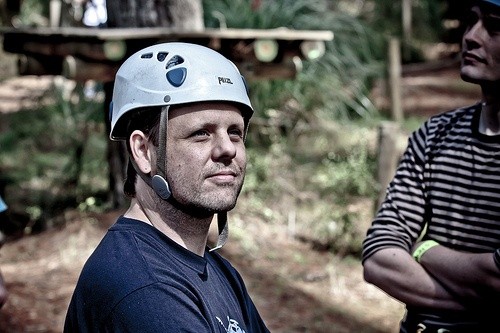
[{"left": 413, "top": 236, "right": 440, "bottom": 265}]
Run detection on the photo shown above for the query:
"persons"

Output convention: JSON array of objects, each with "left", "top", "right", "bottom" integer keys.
[
  {"left": 361, "top": 1, "right": 500, "bottom": 333},
  {"left": 61, "top": 41, "right": 274, "bottom": 333}
]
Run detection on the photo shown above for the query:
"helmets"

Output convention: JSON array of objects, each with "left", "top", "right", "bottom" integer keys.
[{"left": 109, "top": 42, "right": 254, "bottom": 141}]
